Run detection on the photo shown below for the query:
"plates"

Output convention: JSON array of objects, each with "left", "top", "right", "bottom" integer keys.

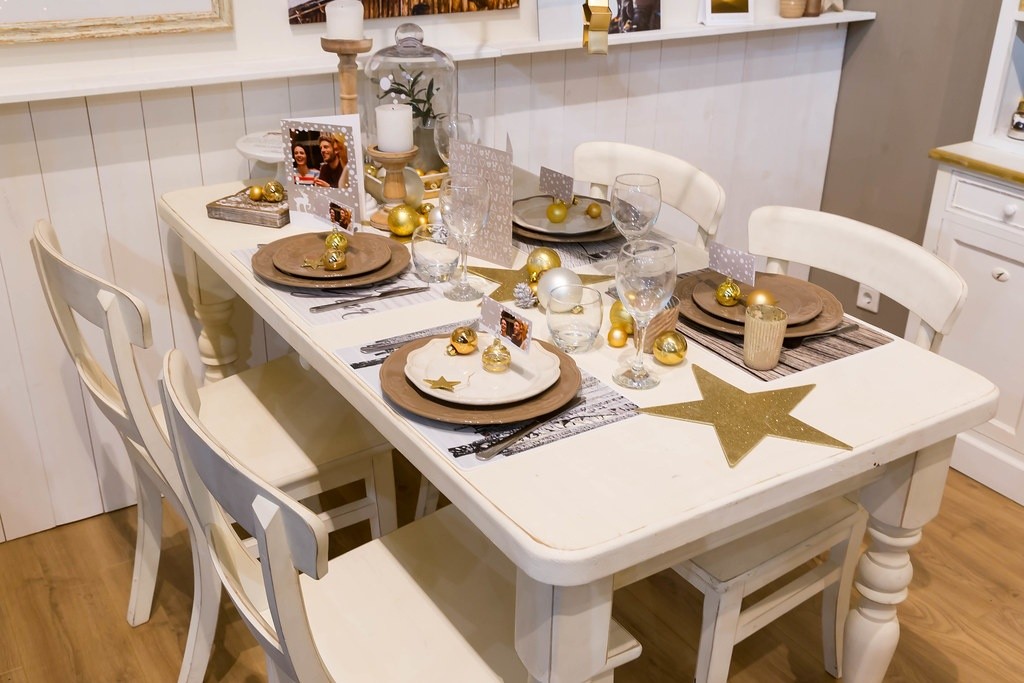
[
  {"left": 380, "top": 331, "right": 581, "bottom": 424},
  {"left": 512, "top": 196, "right": 622, "bottom": 242},
  {"left": 251, "top": 231, "right": 411, "bottom": 288},
  {"left": 675, "top": 270, "right": 843, "bottom": 337}
]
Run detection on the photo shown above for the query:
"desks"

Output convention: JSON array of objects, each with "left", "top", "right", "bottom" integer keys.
[{"left": 151, "top": 149, "right": 998, "bottom": 683}]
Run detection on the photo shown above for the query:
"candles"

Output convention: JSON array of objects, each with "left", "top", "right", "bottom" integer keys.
[
  {"left": 375, "top": 103, "right": 414, "bottom": 152},
  {"left": 325, "top": 0, "right": 364, "bottom": 41}
]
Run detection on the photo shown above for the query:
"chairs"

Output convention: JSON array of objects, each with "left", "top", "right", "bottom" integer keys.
[{"left": 29, "top": 141, "right": 968, "bottom": 683}]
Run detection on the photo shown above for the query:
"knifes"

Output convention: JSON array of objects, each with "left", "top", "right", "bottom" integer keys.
[
  {"left": 310, "top": 287, "right": 431, "bottom": 313},
  {"left": 476, "top": 396, "right": 586, "bottom": 461}
]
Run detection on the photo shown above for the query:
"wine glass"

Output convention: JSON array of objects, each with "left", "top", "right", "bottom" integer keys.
[
  {"left": 440, "top": 175, "right": 490, "bottom": 302},
  {"left": 611, "top": 174, "right": 662, "bottom": 255},
  {"left": 434, "top": 114, "right": 474, "bottom": 168},
  {"left": 613, "top": 239, "right": 677, "bottom": 390}
]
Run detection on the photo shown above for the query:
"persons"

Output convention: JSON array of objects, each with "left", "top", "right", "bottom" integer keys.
[
  {"left": 329, "top": 207, "right": 340, "bottom": 225},
  {"left": 314, "top": 135, "right": 343, "bottom": 188},
  {"left": 511, "top": 319, "right": 527, "bottom": 347},
  {"left": 339, "top": 208, "right": 351, "bottom": 229},
  {"left": 500, "top": 318, "right": 512, "bottom": 341},
  {"left": 292, "top": 143, "right": 320, "bottom": 186}
]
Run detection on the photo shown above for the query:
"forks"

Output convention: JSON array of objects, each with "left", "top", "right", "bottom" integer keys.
[
  {"left": 578, "top": 245, "right": 659, "bottom": 260},
  {"left": 361, "top": 322, "right": 479, "bottom": 354},
  {"left": 782, "top": 324, "right": 858, "bottom": 349}
]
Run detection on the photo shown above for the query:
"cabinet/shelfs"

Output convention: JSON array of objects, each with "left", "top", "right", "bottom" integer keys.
[{"left": 901, "top": 164, "right": 1024, "bottom": 504}]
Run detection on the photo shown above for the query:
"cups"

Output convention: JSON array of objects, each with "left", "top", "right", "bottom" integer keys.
[
  {"left": 744, "top": 305, "right": 788, "bottom": 371},
  {"left": 633, "top": 296, "right": 680, "bottom": 355},
  {"left": 546, "top": 285, "right": 603, "bottom": 354},
  {"left": 412, "top": 224, "right": 460, "bottom": 283}
]
